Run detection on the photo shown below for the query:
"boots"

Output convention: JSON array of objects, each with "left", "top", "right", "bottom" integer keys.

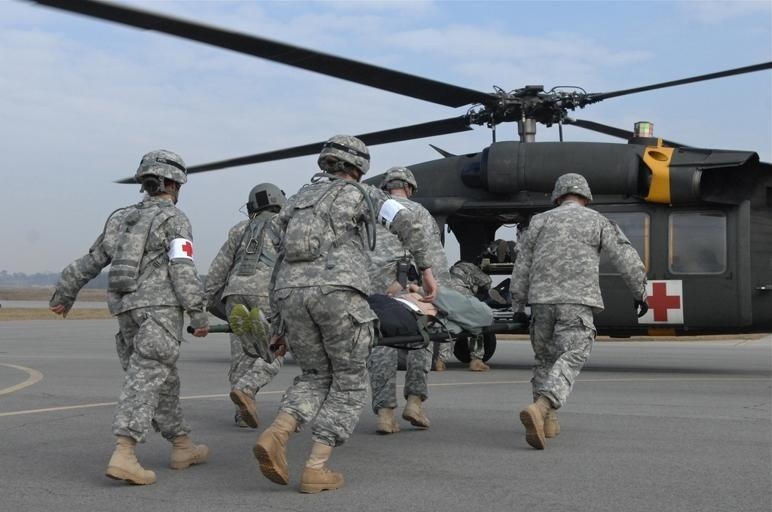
[
  {"left": 106, "top": 434, "right": 157, "bottom": 486},
  {"left": 300, "top": 442, "right": 343, "bottom": 494},
  {"left": 375, "top": 407, "right": 401, "bottom": 432},
  {"left": 520, "top": 395, "right": 560, "bottom": 448},
  {"left": 170, "top": 434, "right": 208, "bottom": 470},
  {"left": 403, "top": 394, "right": 429, "bottom": 428},
  {"left": 255, "top": 409, "right": 296, "bottom": 484}
]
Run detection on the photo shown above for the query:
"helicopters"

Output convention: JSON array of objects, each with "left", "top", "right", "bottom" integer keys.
[{"left": 20, "top": 0, "right": 771, "bottom": 363}]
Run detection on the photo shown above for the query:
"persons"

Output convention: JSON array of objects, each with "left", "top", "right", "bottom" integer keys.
[
  {"left": 253, "top": 136, "right": 436, "bottom": 494},
  {"left": 48, "top": 151, "right": 209, "bottom": 485},
  {"left": 511, "top": 172, "right": 648, "bottom": 450},
  {"left": 204, "top": 167, "right": 451, "bottom": 434},
  {"left": 435, "top": 261, "right": 491, "bottom": 371}
]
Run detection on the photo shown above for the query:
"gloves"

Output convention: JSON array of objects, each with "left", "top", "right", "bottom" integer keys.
[
  {"left": 512, "top": 313, "right": 527, "bottom": 323},
  {"left": 634, "top": 299, "right": 648, "bottom": 317}
]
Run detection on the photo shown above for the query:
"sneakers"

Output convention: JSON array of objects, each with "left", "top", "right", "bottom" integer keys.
[
  {"left": 230, "top": 305, "right": 288, "bottom": 364},
  {"left": 230, "top": 389, "right": 259, "bottom": 427},
  {"left": 432, "top": 359, "right": 447, "bottom": 370},
  {"left": 470, "top": 361, "right": 489, "bottom": 371}
]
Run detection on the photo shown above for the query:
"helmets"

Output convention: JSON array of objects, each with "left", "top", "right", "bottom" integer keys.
[
  {"left": 318, "top": 135, "right": 370, "bottom": 173},
  {"left": 378, "top": 167, "right": 418, "bottom": 190},
  {"left": 247, "top": 183, "right": 287, "bottom": 211},
  {"left": 136, "top": 151, "right": 186, "bottom": 186},
  {"left": 552, "top": 173, "right": 593, "bottom": 201}
]
